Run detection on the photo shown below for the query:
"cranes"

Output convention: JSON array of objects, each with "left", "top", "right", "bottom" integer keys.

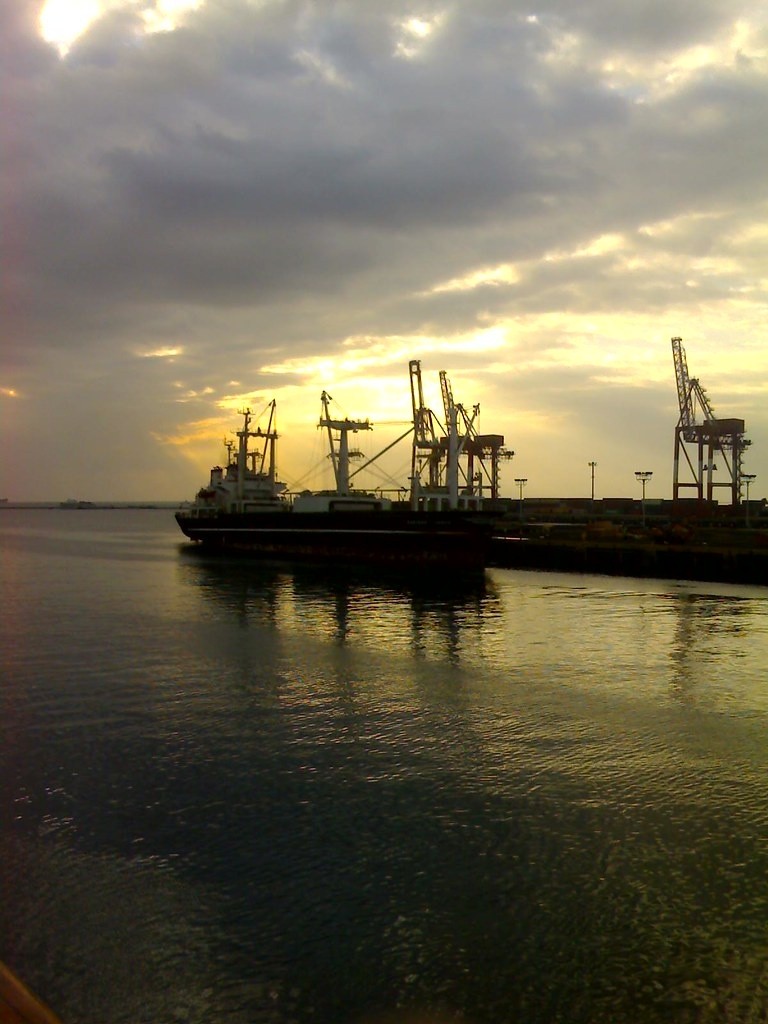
[
  {"left": 668, "top": 336, "right": 753, "bottom": 508},
  {"left": 406, "top": 359, "right": 515, "bottom": 512}
]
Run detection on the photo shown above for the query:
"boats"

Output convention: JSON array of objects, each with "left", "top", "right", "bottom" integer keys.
[{"left": 174, "top": 391, "right": 497, "bottom": 574}]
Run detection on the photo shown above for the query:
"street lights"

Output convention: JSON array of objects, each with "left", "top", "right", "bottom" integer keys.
[
  {"left": 738, "top": 474, "right": 756, "bottom": 528},
  {"left": 514, "top": 478, "right": 527, "bottom": 523},
  {"left": 634, "top": 471, "right": 653, "bottom": 530},
  {"left": 588, "top": 462, "right": 597, "bottom": 500}
]
